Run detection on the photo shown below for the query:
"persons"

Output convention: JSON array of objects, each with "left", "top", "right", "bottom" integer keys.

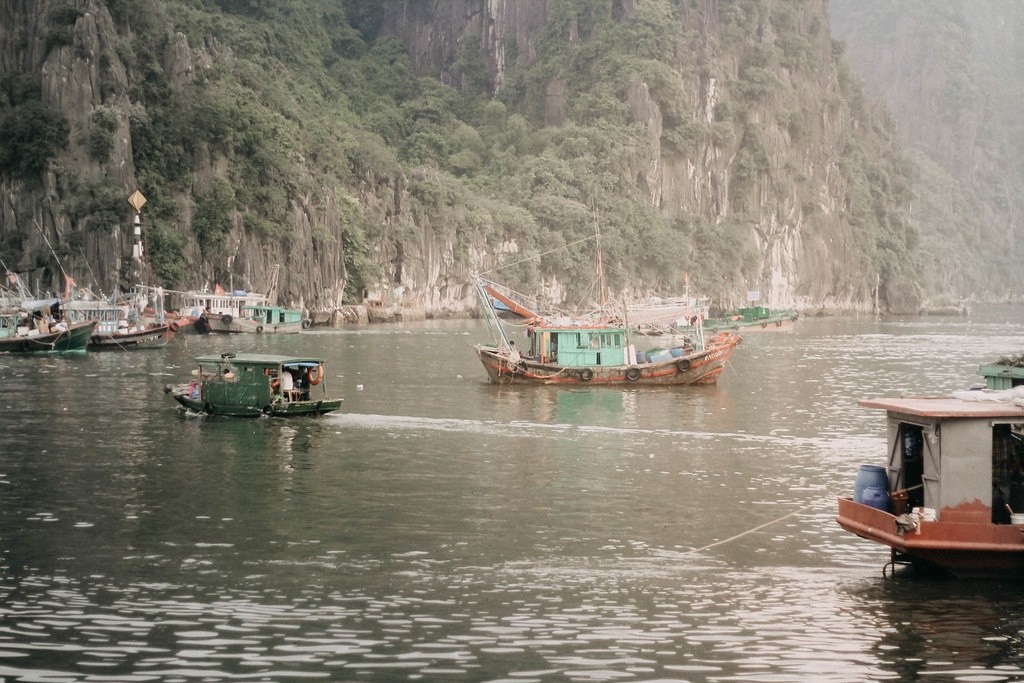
[
  {"left": 223, "top": 364, "right": 311, "bottom": 402},
  {"left": 510, "top": 340, "right": 520, "bottom": 362}
]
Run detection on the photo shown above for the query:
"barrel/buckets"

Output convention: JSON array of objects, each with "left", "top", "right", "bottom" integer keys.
[
  {"left": 636, "top": 350, "right": 646, "bottom": 364},
  {"left": 853, "top": 465, "right": 889, "bottom": 511}
]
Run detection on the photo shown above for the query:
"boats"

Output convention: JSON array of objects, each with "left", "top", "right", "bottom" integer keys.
[
  {"left": 0, "top": 296, "right": 100, "bottom": 352},
  {"left": 693, "top": 300, "right": 810, "bottom": 331},
  {"left": 465, "top": 209, "right": 743, "bottom": 391},
  {"left": 90, "top": 319, "right": 179, "bottom": 350},
  {"left": 199, "top": 300, "right": 311, "bottom": 335},
  {"left": 832, "top": 349, "right": 1024, "bottom": 573},
  {"left": 162, "top": 353, "right": 345, "bottom": 418}
]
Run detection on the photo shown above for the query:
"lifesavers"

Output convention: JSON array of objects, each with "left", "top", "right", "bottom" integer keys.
[
  {"left": 580, "top": 368, "right": 594, "bottom": 381},
  {"left": 303, "top": 320, "right": 311, "bottom": 329},
  {"left": 514, "top": 361, "right": 528, "bottom": 375},
  {"left": 263, "top": 405, "right": 273, "bottom": 415},
  {"left": 222, "top": 315, "right": 233, "bottom": 325},
  {"left": 257, "top": 325, "right": 264, "bottom": 333},
  {"left": 205, "top": 401, "right": 213, "bottom": 415},
  {"left": 169, "top": 322, "right": 179, "bottom": 333},
  {"left": 676, "top": 357, "right": 692, "bottom": 373},
  {"left": 626, "top": 366, "right": 641, "bottom": 381},
  {"left": 308, "top": 365, "right": 323, "bottom": 385}
]
